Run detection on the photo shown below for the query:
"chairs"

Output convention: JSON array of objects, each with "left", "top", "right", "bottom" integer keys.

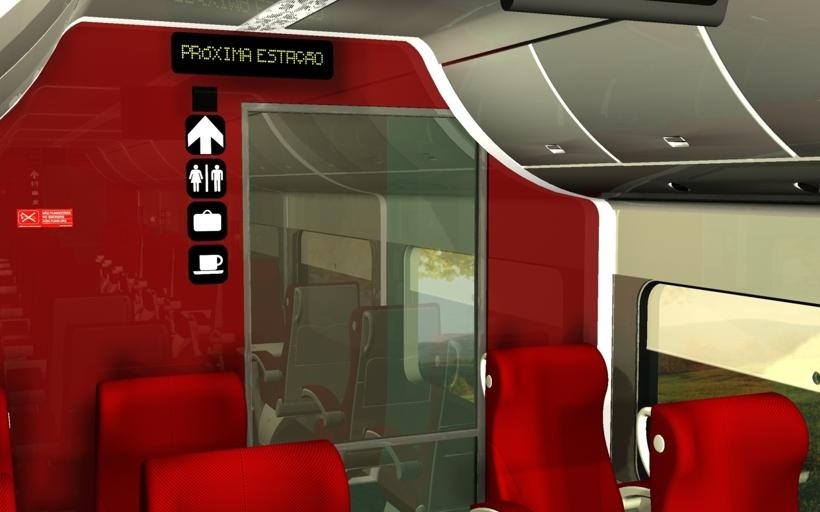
[
  {"left": 480, "top": 346, "right": 625, "bottom": 511},
  {"left": 250, "top": 280, "right": 477, "bottom": 510},
  {"left": 90, "top": 369, "right": 353, "bottom": 511},
  {"left": 634, "top": 392, "right": 813, "bottom": 511}
]
